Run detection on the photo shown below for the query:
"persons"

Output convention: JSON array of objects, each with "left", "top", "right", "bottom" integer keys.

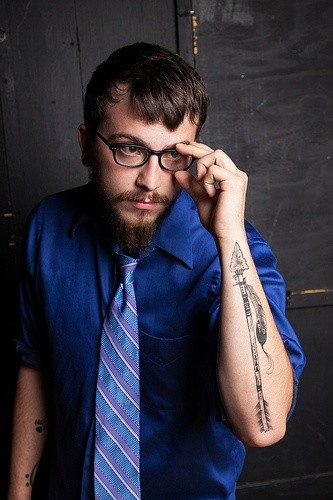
[{"left": 3, "top": 40, "right": 310, "bottom": 500}]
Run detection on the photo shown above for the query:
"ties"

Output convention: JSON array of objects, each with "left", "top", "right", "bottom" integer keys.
[{"left": 88, "top": 244, "right": 156, "bottom": 500}]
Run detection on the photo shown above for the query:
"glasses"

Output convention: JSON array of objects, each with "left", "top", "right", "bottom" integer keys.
[{"left": 85, "top": 126, "right": 196, "bottom": 173}]
